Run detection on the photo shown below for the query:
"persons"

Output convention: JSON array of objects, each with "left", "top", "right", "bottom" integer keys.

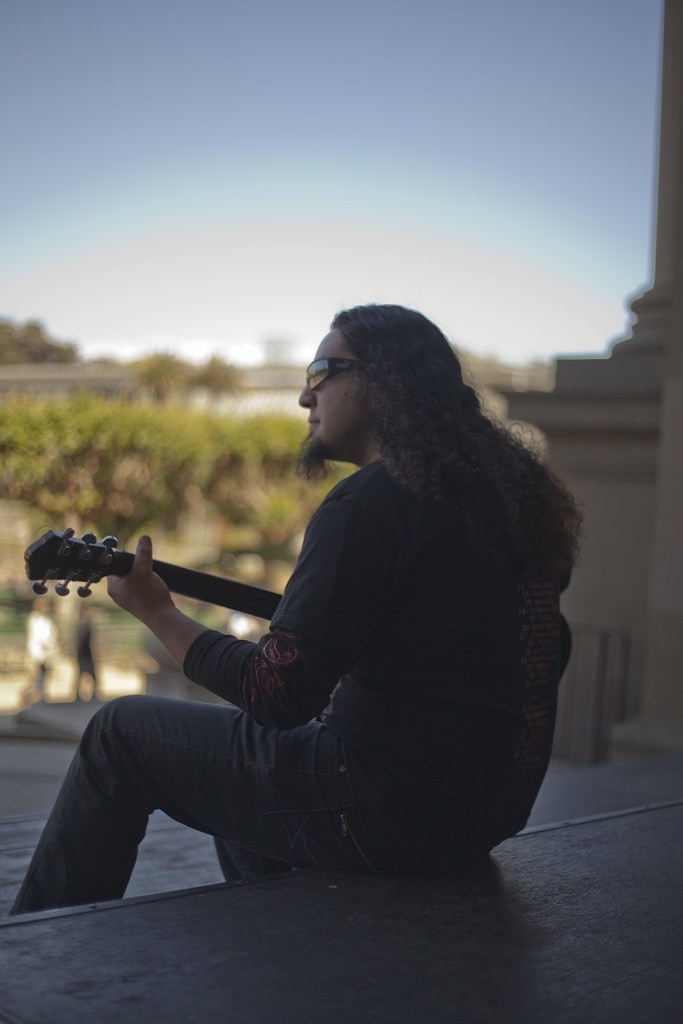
[
  {"left": 74, "top": 606, "right": 100, "bottom": 704},
  {"left": 10, "top": 307, "right": 584, "bottom": 916},
  {"left": 25, "top": 597, "right": 55, "bottom": 704}
]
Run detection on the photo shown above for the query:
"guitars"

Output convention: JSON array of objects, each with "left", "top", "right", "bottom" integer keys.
[{"left": 23, "top": 526, "right": 573, "bottom": 685}]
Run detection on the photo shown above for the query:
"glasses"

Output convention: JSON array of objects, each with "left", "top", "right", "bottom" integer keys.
[{"left": 306, "top": 357, "right": 375, "bottom": 391}]
